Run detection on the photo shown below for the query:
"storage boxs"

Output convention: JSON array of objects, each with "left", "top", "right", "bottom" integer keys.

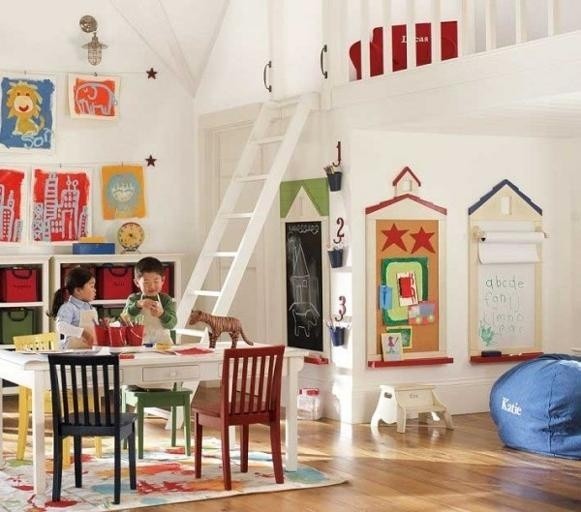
[{"left": 72, "top": 244, "right": 114, "bottom": 255}]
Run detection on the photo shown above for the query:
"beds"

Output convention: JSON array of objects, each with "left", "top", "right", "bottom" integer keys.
[{"left": 328, "top": 1, "right": 581, "bottom": 140}]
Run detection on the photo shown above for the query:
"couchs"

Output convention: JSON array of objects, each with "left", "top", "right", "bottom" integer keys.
[{"left": 349, "top": 22, "right": 458, "bottom": 80}]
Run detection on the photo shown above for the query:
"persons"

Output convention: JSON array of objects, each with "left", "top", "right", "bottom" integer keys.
[
  {"left": 47, "top": 268, "right": 99, "bottom": 349},
  {"left": 121, "top": 257, "right": 177, "bottom": 390}
]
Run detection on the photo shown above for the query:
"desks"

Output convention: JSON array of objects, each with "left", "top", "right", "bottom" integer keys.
[{"left": 0, "top": 342, "right": 308, "bottom": 508}]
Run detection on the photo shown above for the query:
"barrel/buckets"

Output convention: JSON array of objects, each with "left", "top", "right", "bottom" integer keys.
[
  {"left": 94, "top": 324, "right": 108, "bottom": 346},
  {"left": 127, "top": 324, "right": 145, "bottom": 346},
  {"left": 108, "top": 326, "right": 126, "bottom": 348}
]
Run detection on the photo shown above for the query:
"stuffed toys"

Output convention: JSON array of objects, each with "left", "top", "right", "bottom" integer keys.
[{"left": 188, "top": 310, "right": 255, "bottom": 349}]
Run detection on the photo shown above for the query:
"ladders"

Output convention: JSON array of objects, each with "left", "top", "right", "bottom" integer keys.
[{"left": 134, "top": 94, "right": 315, "bottom": 430}]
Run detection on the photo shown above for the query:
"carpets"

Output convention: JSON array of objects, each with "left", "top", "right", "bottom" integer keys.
[{"left": 0, "top": 438, "right": 349, "bottom": 512}]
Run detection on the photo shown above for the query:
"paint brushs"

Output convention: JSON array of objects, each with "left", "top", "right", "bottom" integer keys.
[{"left": 93, "top": 314, "right": 134, "bottom": 325}]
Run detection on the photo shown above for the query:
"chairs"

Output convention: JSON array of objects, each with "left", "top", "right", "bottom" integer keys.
[
  {"left": 12, "top": 332, "right": 101, "bottom": 469},
  {"left": 47, "top": 353, "right": 136, "bottom": 504},
  {"left": 192, "top": 346, "right": 284, "bottom": 490},
  {"left": 121, "top": 330, "right": 192, "bottom": 459}
]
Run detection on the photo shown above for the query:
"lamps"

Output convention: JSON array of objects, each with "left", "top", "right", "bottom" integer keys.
[{"left": 79, "top": 15, "right": 107, "bottom": 64}]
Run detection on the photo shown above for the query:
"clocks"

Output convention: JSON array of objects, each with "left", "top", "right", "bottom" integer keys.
[{"left": 117, "top": 223, "right": 144, "bottom": 254}]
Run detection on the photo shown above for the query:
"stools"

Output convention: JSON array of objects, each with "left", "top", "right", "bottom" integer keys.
[{"left": 370, "top": 384, "right": 453, "bottom": 433}]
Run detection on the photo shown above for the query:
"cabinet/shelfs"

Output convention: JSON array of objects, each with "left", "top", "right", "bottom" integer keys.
[
  {"left": 49, "top": 254, "right": 182, "bottom": 345},
  {"left": 0, "top": 257, "right": 50, "bottom": 395}
]
